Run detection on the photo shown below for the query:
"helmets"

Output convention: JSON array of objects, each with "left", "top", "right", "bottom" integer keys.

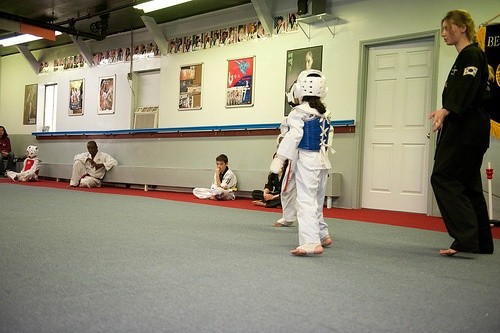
[
  {"left": 27, "top": 146, "right": 39, "bottom": 158},
  {"left": 293, "top": 69, "right": 329, "bottom": 101},
  {"left": 286, "top": 80, "right": 301, "bottom": 109}
]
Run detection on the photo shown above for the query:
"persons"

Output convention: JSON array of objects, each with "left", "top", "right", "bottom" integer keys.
[
  {"left": 39, "top": 13, "right": 297, "bottom": 73},
  {"left": 0, "top": 126, "right": 14, "bottom": 177},
  {"left": 70, "top": 140, "right": 118, "bottom": 189},
  {"left": 193, "top": 154, "right": 238, "bottom": 200},
  {"left": 285, "top": 50, "right": 314, "bottom": 110},
  {"left": 253, "top": 68, "right": 333, "bottom": 256},
  {"left": 6, "top": 144, "right": 42, "bottom": 182},
  {"left": 427, "top": 7, "right": 494, "bottom": 256}
]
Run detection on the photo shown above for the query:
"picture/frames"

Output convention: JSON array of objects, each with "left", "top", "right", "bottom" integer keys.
[
  {"left": 97, "top": 74, "right": 116, "bottom": 115},
  {"left": 68, "top": 78, "right": 85, "bottom": 116},
  {"left": 23, "top": 83, "right": 38, "bottom": 125},
  {"left": 225, "top": 55, "right": 256, "bottom": 109},
  {"left": 284, "top": 45, "right": 323, "bottom": 116},
  {"left": 177, "top": 62, "right": 204, "bottom": 111}
]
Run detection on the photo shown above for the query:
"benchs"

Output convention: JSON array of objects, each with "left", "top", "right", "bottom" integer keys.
[{"left": 2, "top": 159, "right": 342, "bottom": 209}]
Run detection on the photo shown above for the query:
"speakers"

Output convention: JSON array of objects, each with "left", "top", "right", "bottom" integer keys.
[{"left": 297, "top": 0, "right": 325, "bottom": 18}]
